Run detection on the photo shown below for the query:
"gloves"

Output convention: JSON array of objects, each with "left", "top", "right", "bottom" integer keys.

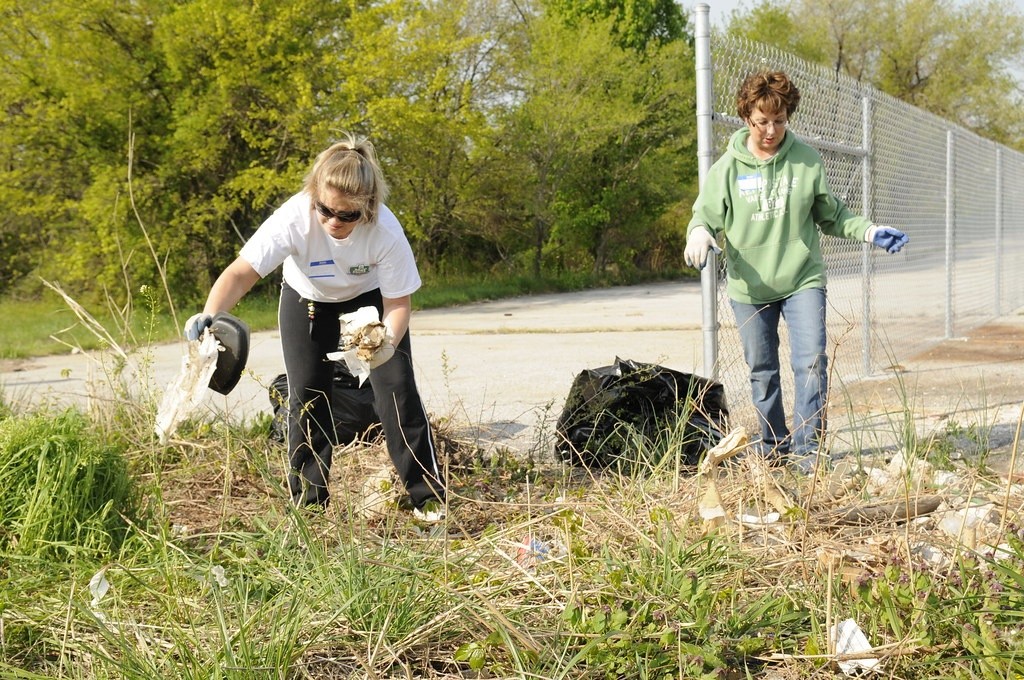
[
  {"left": 684, "top": 225, "right": 723, "bottom": 271},
  {"left": 183, "top": 313, "right": 212, "bottom": 341},
  {"left": 370, "top": 343, "right": 395, "bottom": 369},
  {"left": 864, "top": 225, "right": 909, "bottom": 254}
]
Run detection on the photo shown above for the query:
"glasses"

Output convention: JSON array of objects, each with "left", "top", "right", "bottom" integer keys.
[
  {"left": 314, "top": 201, "right": 362, "bottom": 222},
  {"left": 749, "top": 115, "right": 790, "bottom": 129}
]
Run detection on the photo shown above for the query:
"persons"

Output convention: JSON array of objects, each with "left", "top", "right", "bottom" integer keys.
[
  {"left": 184, "top": 132, "right": 453, "bottom": 523},
  {"left": 683, "top": 70, "right": 909, "bottom": 475}
]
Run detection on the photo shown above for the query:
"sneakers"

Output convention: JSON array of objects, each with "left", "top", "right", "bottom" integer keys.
[
  {"left": 414, "top": 497, "right": 446, "bottom": 526},
  {"left": 802, "top": 453, "right": 832, "bottom": 477}
]
